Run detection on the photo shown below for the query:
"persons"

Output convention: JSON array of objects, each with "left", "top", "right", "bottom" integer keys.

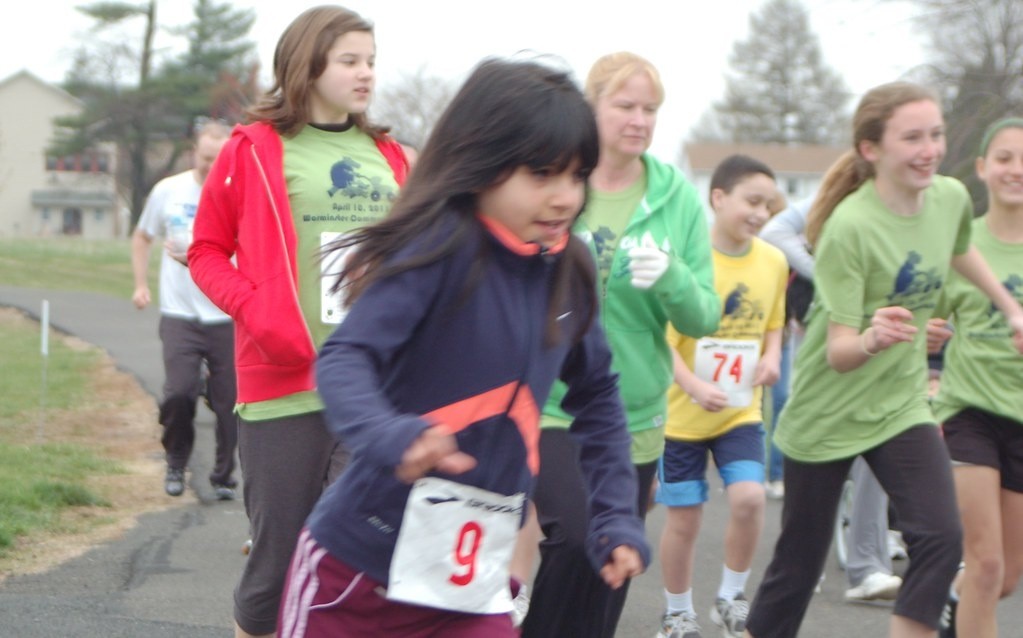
[
  {"left": 129, "top": 122, "right": 239, "bottom": 502},
  {"left": 927, "top": 117, "right": 1021, "bottom": 636},
  {"left": 517, "top": 51, "right": 721, "bottom": 638},
  {"left": 277, "top": 58, "right": 652, "bottom": 638},
  {"left": 743, "top": 80, "right": 1022, "bottom": 636},
  {"left": 759, "top": 194, "right": 900, "bottom": 604},
  {"left": 656, "top": 155, "right": 790, "bottom": 638},
  {"left": 186, "top": 5, "right": 409, "bottom": 638}
]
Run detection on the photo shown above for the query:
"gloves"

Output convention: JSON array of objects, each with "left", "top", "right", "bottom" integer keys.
[{"left": 628, "top": 233, "right": 669, "bottom": 289}]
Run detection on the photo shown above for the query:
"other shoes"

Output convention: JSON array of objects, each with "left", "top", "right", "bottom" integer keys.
[
  {"left": 887, "top": 531, "right": 907, "bottom": 559},
  {"left": 765, "top": 480, "right": 785, "bottom": 498}
]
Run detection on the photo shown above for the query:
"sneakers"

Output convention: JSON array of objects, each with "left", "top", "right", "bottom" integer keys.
[
  {"left": 214, "top": 484, "right": 236, "bottom": 500},
  {"left": 656, "top": 612, "right": 702, "bottom": 638},
  {"left": 709, "top": 592, "right": 749, "bottom": 638},
  {"left": 165, "top": 465, "right": 184, "bottom": 496},
  {"left": 936, "top": 560, "right": 965, "bottom": 638},
  {"left": 845, "top": 572, "right": 903, "bottom": 600}
]
